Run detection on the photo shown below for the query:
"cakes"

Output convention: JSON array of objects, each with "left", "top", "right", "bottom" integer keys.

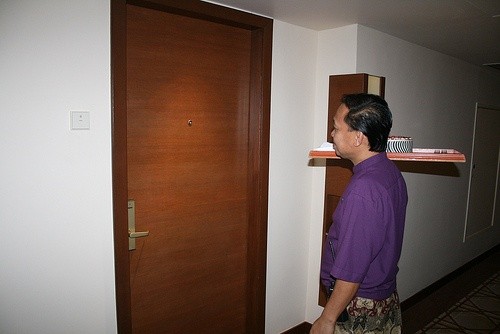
[{"left": 385, "top": 136, "right": 413, "bottom": 153}]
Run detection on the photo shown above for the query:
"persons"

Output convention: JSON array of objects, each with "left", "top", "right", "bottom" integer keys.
[{"left": 310, "top": 93, "right": 409, "bottom": 334}]
